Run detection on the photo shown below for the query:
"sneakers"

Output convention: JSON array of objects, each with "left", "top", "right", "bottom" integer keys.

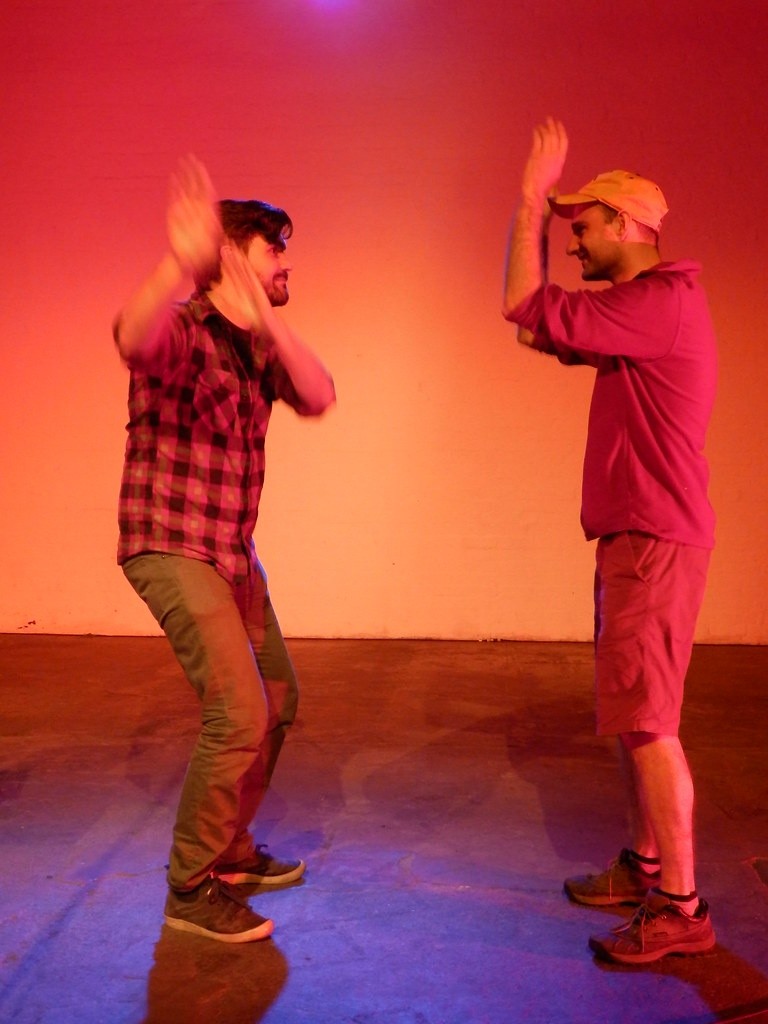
[
  {"left": 163, "top": 878, "right": 275, "bottom": 943},
  {"left": 563, "top": 857, "right": 660, "bottom": 904},
  {"left": 589, "top": 888, "right": 716, "bottom": 964},
  {"left": 210, "top": 843, "right": 304, "bottom": 887}
]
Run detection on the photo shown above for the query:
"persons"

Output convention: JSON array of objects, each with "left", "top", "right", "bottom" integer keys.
[
  {"left": 501, "top": 116, "right": 717, "bottom": 963},
  {"left": 112, "top": 150, "right": 337, "bottom": 944}
]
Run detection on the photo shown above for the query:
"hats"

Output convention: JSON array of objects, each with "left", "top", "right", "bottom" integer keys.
[{"left": 550, "top": 169, "right": 669, "bottom": 233}]
